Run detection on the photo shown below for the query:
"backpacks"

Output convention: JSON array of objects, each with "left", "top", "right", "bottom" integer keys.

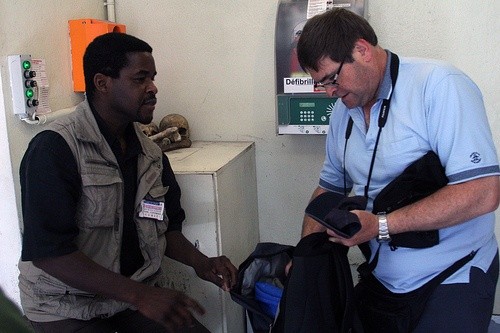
[{"left": 224, "top": 230, "right": 363, "bottom": 333}]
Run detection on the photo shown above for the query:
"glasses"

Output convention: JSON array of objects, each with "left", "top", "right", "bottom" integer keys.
[{"left": 313, "top": 56, "right": 346, "bottom": 91}]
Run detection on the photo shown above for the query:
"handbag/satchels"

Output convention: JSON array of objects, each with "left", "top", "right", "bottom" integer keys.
[{"left": 372, "top": 150, "right": 450, "bottom": 250}]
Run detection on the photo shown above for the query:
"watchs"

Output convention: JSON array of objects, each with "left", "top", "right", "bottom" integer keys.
[{"left": 377, "top": 213, "right": 391, "bottom": 245}]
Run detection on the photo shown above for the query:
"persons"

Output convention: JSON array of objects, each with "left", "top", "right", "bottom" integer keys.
[
  {"left": 18, "top": 31, "right": 239, "bottom": 333},
  {"left": 284, "top": 6, "right": 500, "bottom": 333}
]
